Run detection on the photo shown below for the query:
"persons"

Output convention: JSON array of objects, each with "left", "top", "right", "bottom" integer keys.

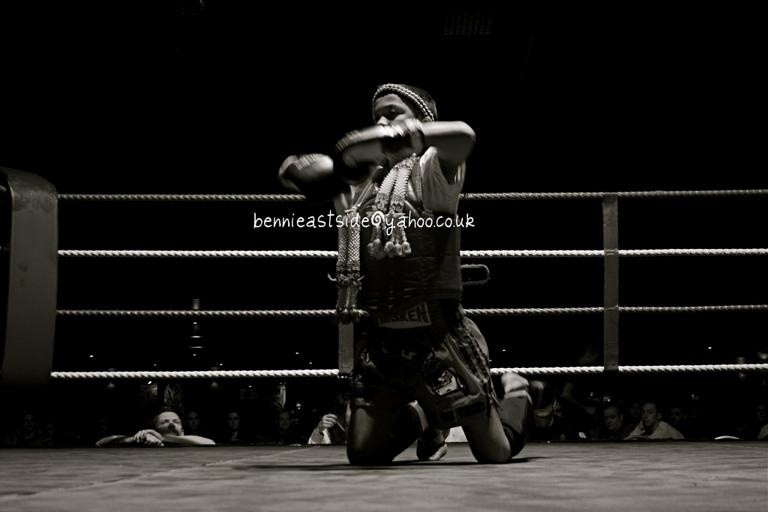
[{"left": 276, "top": 78, "right": 532, "bottom": 462}]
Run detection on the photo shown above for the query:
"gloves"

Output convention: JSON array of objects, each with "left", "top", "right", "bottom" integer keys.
[
  {"left": 278, "top": 152, "right": 333, "bottom": 200},
  {"left": 337, "top": 119, "right": 426, "bottom": 184}
]
